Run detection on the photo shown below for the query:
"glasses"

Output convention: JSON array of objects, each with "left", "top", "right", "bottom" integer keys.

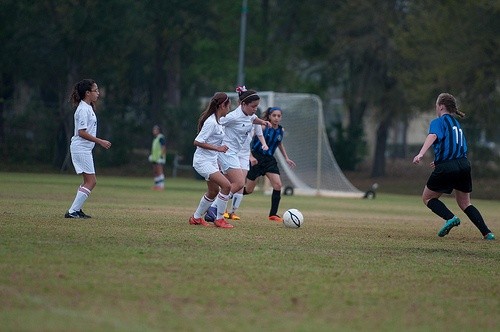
[{"left": 91, "top": 89, "right": 99, "bottom": 93}]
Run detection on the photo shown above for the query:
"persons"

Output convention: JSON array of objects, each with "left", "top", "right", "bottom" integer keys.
[
  {"left": 189, "top": 85, "right": 295, "bottom": 228},
  {"left": 64, "top": 79, "right": 111, "bottom": 218},
  {"left": 412, "top": 93, "right": 496, "bottom": 240},
  {"left": 150, "top": 125, "right": 166, "bottom": 191}
]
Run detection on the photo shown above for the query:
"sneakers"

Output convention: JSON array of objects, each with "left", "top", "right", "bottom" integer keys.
[
  {"left": 269, "top": 215, "right": 282, "bottom": 222},
  {"left": 224, "top": 212, "right": 230, "bottom": 218},
  {"left": 205, "top": 207, "right": 217, "bottom": 222},
  {"left": 65, "top": 211, "right": 87, "bottom": 219},
  {"left": 189, "top": 216, "right": 213, "bottom": 227},
  {"left": 214, "top": 218, "right": 233, "bottom": 228},
  {"left": 484, "top": 233, "right": 495, "bottom": 240},
  {"left": 80, "top": 209, "right": 91, "bottom": 218},
  {"left": 438, "top": 215, "right": 461, "bottom": 237},
  {"left": 230, "top": 213, "right": 240, "bottom": 220}
]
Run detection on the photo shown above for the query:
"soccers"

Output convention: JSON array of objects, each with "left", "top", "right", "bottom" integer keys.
[{"left": 283, "top": 209, "right": 303, "bottom": 228}]
